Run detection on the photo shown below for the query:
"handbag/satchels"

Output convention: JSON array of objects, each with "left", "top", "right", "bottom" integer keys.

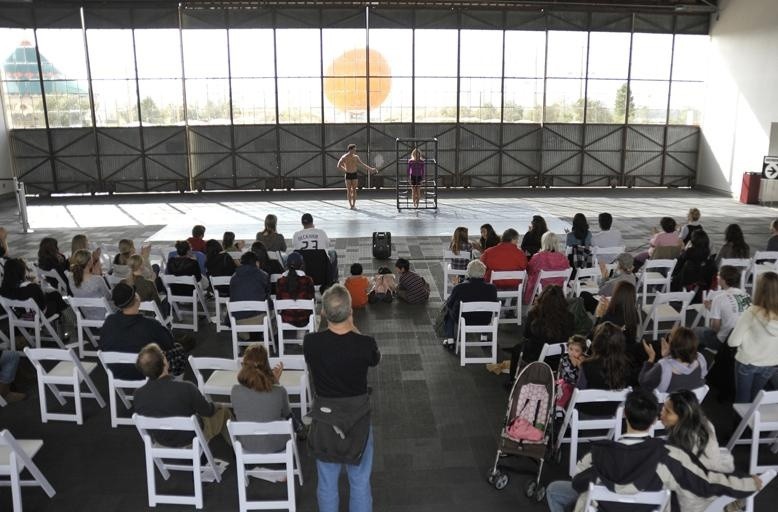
[{"left": 435, "top": 301, "right": 449, "bottom": 337}]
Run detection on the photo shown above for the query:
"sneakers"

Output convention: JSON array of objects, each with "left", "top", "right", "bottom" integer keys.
[{"left": 0, "top": 392, "right": 26, "bottom": 401}]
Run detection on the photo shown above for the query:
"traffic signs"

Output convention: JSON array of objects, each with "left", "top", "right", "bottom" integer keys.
[{"left": 765, "top": 156, "right": 778, "bottom": 178}]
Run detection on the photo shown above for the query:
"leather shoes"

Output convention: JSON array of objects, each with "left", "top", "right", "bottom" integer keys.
[
  {"left": 480, "top": 340, "right": 488, "bottom": 348},
  {"left": 443, "top": 339, "right": 455, "bottom": 350},
  {"left": 556, "top": 411, "right": 565, "bottom": 423}
]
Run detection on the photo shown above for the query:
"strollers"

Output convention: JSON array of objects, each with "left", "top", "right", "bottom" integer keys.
[{"left": 487, "top": 337, "right": 565, "bottom": 502}]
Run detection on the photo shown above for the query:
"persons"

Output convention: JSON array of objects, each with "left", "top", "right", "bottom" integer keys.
[
  {"left": 366, "top": 266, "right": 398, "bottom": 304},
  {"left": 1, "top": 212, "right": 339, "bottom": 472},
  {"left": 302, "top": 283, "right": 382, "bottom": 512},
  {"left": 394, "top": 256, "right": 431, "bottom": 308},
  {"left": 434, "top": 205, "right": 778, "bottom": 512},
  {"left": 407, "top": 146, "right": 426, "bottom": 209},
  {"left": 336, "top": 142, "right": 379, "bottom": 210},
  {"left": 342, "top": 262, "right": 373, "bottom": 311}
]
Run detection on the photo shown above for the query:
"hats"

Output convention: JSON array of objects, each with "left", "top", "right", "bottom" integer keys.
[{"left": 112, "top": 283, "right": 135, "bottom": 307}]
